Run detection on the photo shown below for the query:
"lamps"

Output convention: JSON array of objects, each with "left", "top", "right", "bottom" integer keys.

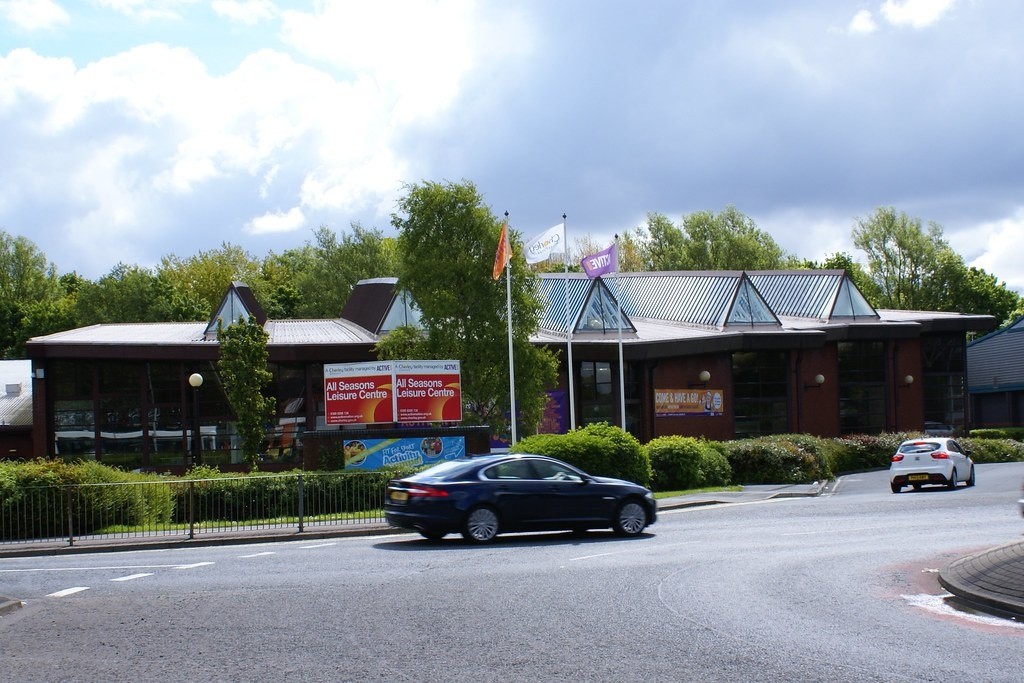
[
  {"left": 900, "top": 375, "right": 913, "bottom": 388},
  {"left": 804, "top": 374, "right": 825, "bottom": 388},
  {"left": 689, "top": 371, "right": 711, "bottom": 389}
]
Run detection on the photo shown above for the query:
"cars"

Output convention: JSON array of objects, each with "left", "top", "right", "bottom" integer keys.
[
  {"left": 887, "top": 437, "right": 976, "bottom": 493},
  {"left": 382, "top": 453, "right": 658, "bottom": 546}
]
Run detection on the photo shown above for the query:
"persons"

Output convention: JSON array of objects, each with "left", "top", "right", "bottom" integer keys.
[
  {"left": 345, "top": 442, "right": 365, "bottom": 461},
  {"left": 422, "top": 439, "right": 441, "bottom": 455}
]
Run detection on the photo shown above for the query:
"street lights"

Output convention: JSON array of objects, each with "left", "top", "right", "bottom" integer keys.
[{"left": 190, "top": 371, "right": 203, "bottom": 479}]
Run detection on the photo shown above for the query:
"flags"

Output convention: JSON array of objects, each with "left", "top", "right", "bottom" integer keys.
[
  {"left": 493, "top": 216, "right": 515, "bottom": 280},
  {"left": 581, "top": 243, "right": 617, "bottom": 280},
  {"left": 521, "top": 223, "right": 567, "bottom": 264}
]
